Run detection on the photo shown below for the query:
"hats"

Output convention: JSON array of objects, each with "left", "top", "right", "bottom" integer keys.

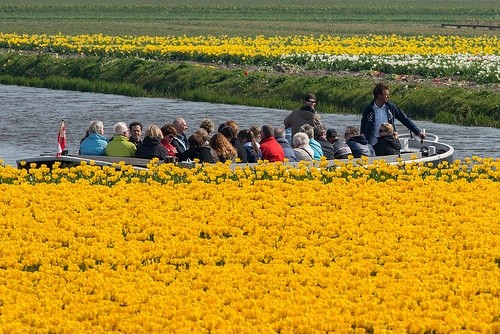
[
  {"left": 218, "top": 124, "right": 230, "bottom": 133},
  {"left": 314, "top": 125, "right": 325, "bottom": 133},
  {"left": 326, "top": 128, "right": 337, "bottom": 138},
  {"left": 113, "top": 123, "right": 128, "bottom": 134},
  {"left": 305, "top": 93, "right": 316, "bottom": 103}
]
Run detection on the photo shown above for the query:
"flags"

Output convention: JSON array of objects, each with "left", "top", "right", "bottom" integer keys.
[{"left": 57, "top": 121, "right": 66, "bottom": 157}]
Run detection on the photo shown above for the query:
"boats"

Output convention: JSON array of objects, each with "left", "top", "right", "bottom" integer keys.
[{"left": 15, "top": 127, "right": 455, "bottom": 177}]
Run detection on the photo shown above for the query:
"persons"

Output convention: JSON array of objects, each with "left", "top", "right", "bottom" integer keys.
[
  {"left": 284, "top": 92, "right": 322, "bottom": 135},
  {"left": 79, "top": 120, "right": 143, "bottom": 158},
  {"left": 360, "top": 82, "right": 426, "bottom": 148},
  {"left": 313, "top": 123, "right": 376, "bottom": 160},
  {"left": 292, "top": 124, "right": 323, "bottom": 162},
  {"left": 183, "top": 118, "right": 295, "bottom": 163},
  {"left": 372, "top": 122, "right": 401, "bottom": 156},
  {"left": 134, "top": 117, "right": 188, "bottom": 160}
]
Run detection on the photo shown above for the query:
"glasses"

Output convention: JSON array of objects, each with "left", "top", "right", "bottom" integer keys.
[
  {"left": 169, "top": 135, "right": 175, "bottom": 140},
  {"left": 260, "top": 131, "right": 263, "bottom": 133},
  {"left": 345, "top": 133, "right": 353, "bottom": 137},
  {"left": 379, "top": 129, "right": 385, "bottom": 133}
]
